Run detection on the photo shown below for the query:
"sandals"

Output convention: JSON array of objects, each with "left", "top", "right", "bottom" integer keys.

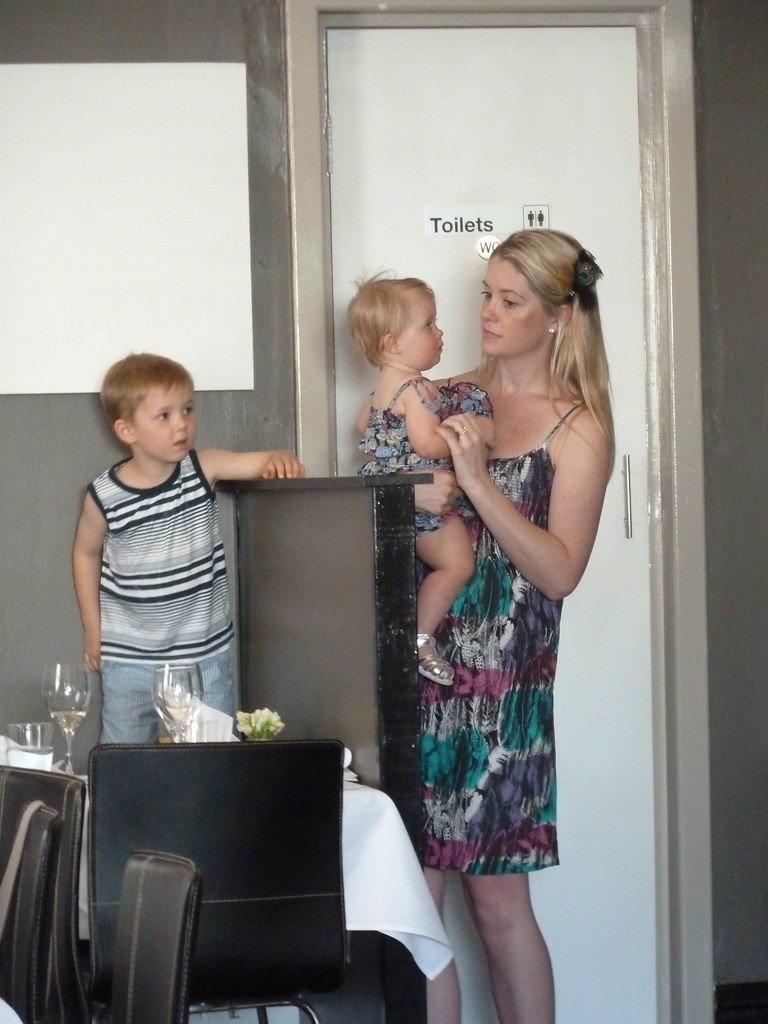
[{"left": 416, "top": 633, "right": 455, "bottom": 685}]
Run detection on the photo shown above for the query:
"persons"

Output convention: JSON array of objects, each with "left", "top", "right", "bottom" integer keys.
[
  {"left": 348, "top": 276, "right": 496, "bottom": 687},
  {"left": 424, "top": 229, "right": 616, "bottom": 1023},
  {"left": 73, "top": 353, "right": 306, "bottom": 745}
]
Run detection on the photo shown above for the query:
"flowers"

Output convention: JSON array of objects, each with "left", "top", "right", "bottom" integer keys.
[{"left": 236, "top": 708, "right": 285, "bottom": 741}]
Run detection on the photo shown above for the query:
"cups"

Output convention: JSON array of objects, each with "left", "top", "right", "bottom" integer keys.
[{"left": 6, "top": 722, "right": 53, "bottom": 754}]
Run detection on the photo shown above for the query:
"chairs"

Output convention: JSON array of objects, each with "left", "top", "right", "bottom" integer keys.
[{"left": 0, "top": 740, "right": 353, "bottom": 1024}]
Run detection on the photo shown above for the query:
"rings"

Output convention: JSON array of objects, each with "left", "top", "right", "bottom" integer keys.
[{"left": 457, "top": 427, "right": 466, "bottom": 436}]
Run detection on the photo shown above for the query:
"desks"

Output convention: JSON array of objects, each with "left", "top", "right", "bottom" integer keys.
[{"left": 77, "top": 778, "right": 453, "bottom": 1024}]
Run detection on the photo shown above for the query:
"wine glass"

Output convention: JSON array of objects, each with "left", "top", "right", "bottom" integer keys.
[
  {"left": 152, "top": 662, "right": 204, "bottom": 743},
  {"left": 41, "top": 664, "right": 92, "bottom": 777}
]
